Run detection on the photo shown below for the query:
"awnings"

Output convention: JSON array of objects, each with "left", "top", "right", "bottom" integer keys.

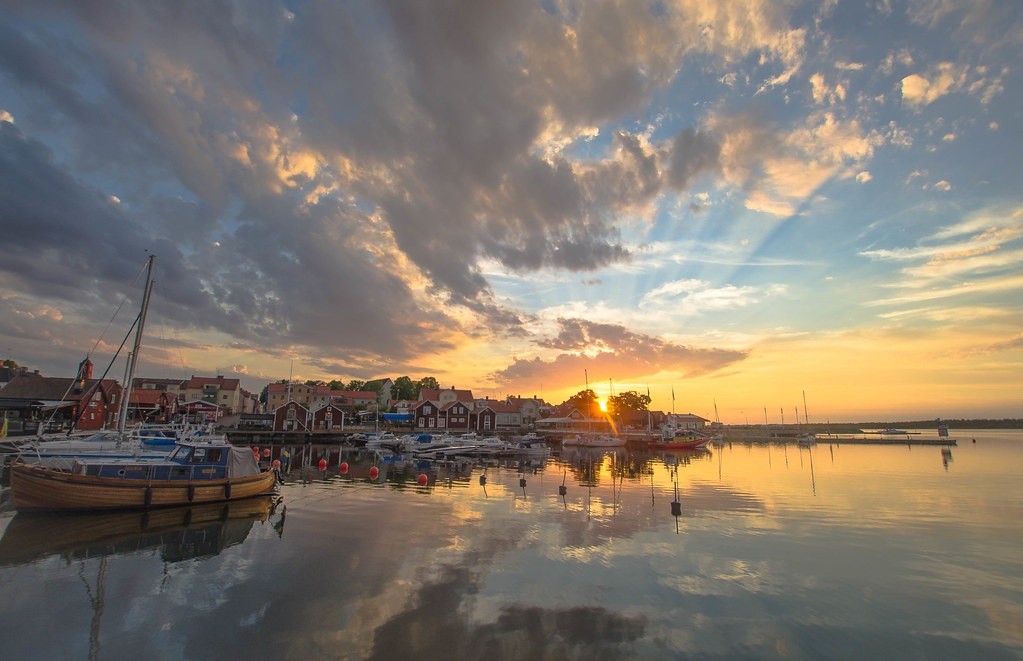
[{"left": 0, "top": 401, "right": 78, "bottom": 412}]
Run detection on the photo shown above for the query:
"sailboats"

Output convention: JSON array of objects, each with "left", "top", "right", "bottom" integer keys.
[{"left": 560, "top": 369, "right": 629, "bottom": 449}]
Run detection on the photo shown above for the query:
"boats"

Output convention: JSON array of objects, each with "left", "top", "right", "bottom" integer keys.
[
  {"left": 8, "top": 433, "right": 281, "bottom": 513},
  {"left": 6, "top": 247, "right": 228, "bottom": 468},
  {"left": 796, "top": 432, "right": 816, "bottom": 443},
  {"left": 0, "top": 491, "right": 282, "bottom": 568},
  {"left": 345, "top": 427, "right": 551, "bottom": 464},
  {"left": 646, "top": 427, "right": 713, "bottom": 449}
]
